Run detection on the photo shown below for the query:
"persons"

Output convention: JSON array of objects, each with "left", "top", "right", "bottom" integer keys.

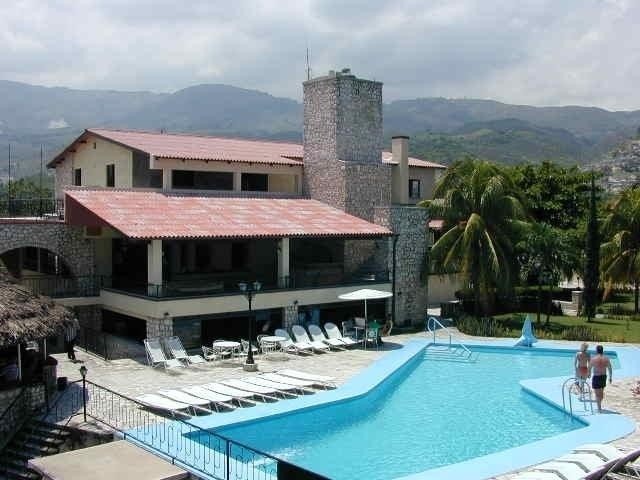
[
  {"left": 575, "top": 343, "right": 591, "bottom": 401},
  {"left": 0, "top": 356, "right": 18, "bottom": 383},
  {"left": 588, "top": 345, "right": 612, "bottom": 413},
  {"left": 63, "top": 319, "right": 81, "bottom": 363}
]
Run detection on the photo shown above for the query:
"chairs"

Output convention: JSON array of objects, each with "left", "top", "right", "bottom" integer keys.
[{"left": 135, "top": 318, "right": 393, "bottom": 424}]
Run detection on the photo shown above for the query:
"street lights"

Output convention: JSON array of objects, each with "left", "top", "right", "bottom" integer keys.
[
  {"left": 389, "top": 232, "right": 402, "bottom": 328},
  {"left": 78, "top": 364, "right": 90, "bottom": 423},
  {"left": 237, "top": 278, "right": 263, "bottom": 365}
]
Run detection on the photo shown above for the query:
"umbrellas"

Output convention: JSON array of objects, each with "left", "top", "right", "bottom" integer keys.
[{"left": 338, "top": 289, "right": 393, "bottom": 321}]
